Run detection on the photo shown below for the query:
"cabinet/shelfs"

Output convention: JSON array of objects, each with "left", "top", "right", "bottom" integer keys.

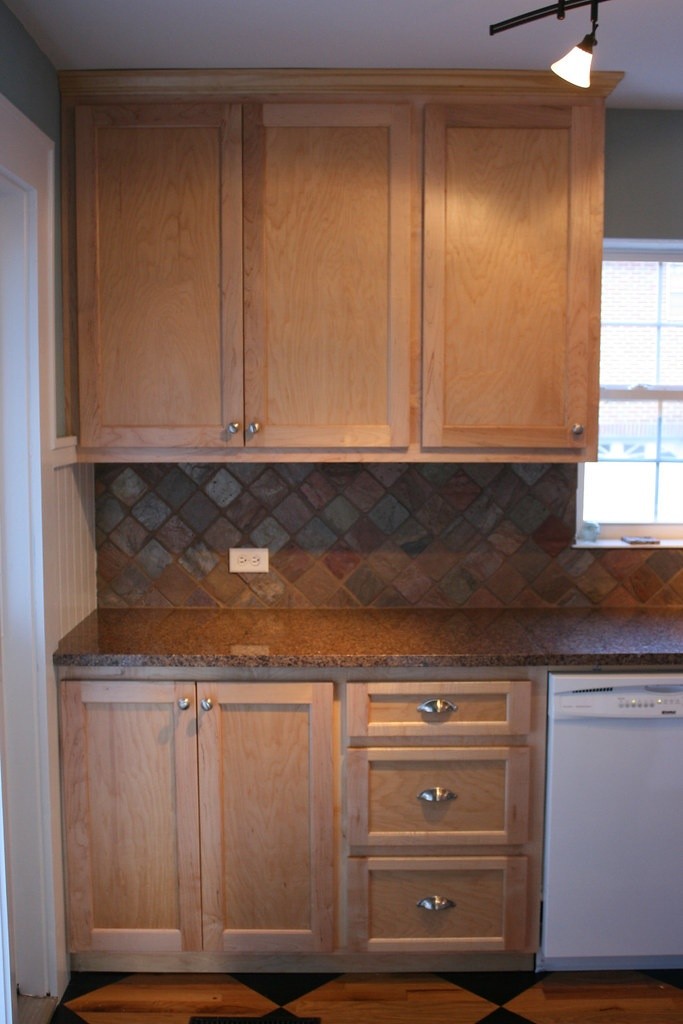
[
  {"left": 402, "top": 72, "right": 621, "bottom": 464},
  {"left": 345, "top": 666, "right": 543, "bottom": 978},
  {"left": 56, "top": 68, "right": 413, "bottom": 465},
  {"left": 59, "top": 672, "right": 338, "bottom": 971}
]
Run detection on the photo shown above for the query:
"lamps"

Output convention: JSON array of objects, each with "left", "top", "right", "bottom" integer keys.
[{"left": 545, "top": 0, "right": 601, "bottom": 91}]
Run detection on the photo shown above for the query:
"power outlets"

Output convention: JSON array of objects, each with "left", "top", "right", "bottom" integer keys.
[{"left": 228, "top": 547, "right": 270, "bottom": 574}]
